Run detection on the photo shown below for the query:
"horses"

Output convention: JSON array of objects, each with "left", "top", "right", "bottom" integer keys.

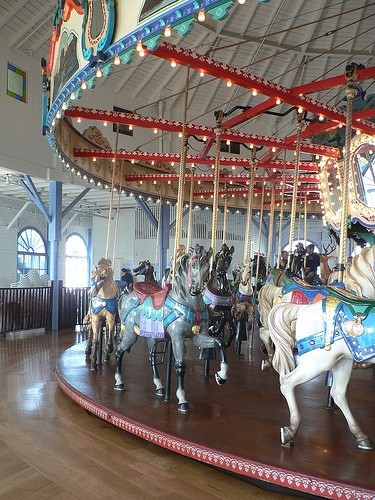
[{"left": 81, "top": 242, "right": 375, "bottom": 451}]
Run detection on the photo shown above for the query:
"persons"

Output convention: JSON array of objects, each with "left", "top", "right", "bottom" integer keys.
[
  {"left": 139, "top": 261, "right": 154, "bottom": 281},
  {"left": 162, "top": 268, "right": 170, "bottom": 288},
  {"left": 305, "top": 244, "right": 320, "bottom": 282},
  {"left": 273, "top": 251, "right": 289, "bottom": 270},
  {"left": 120, "top": 268, "right": 133, "bottom": 285}
]
